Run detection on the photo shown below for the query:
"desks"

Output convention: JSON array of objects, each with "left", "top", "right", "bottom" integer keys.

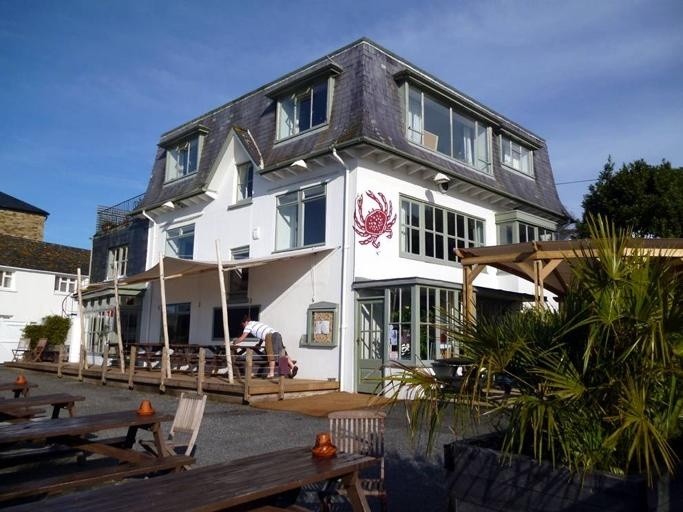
[
  {"left": 1, "top": 441, "right": 375, "bottom": 509},
  {"left": 104, "top": 342, "right": 285, "bottom": 379},
  {"left": 3, "top": 379, "right": 177, "bottom": 474}
]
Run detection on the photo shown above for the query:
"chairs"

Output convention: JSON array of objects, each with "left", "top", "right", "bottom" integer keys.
[
  {"left": 10, "top": 336, "right": 47, "bottom": 363},
  {"left": 139, "top": 391, "right": 207, "bottom": 470},
  {"left": 318, "top": 409, "right": 390, "bottom": 511}
]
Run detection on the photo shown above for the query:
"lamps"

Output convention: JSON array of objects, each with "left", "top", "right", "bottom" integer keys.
[
  {"left": 160, "top": 201, "right": 174, "bottom": 209},
  {"left": 289, "top": 159, "right": 307, "bottom": 170},
  {"left": 433, "top": 172, "right": 450, "bottom": 185}
]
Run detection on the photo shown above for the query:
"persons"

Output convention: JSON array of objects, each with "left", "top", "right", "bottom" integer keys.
[{"left": 233, "top": 314, "right": 298, "bottom": 379}]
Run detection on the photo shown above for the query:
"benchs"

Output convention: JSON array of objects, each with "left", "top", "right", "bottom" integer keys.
[{"left": 1, "top": 453, "right": 197, "bottom": 502}]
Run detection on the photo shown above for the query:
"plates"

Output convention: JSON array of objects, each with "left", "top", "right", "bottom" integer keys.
[
  {"left": 15, "top": 380, "right": 27, "bottom": 385},
  {"left": 310, "top": 444, "right": 338, "bottom": 458},
  {"left": 136, "top": 409, "right": 155, "bottom": 416}
]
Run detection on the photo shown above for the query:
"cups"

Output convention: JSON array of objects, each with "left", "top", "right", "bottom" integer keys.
[
  {"left": 139, "top": 399, "right": 152, "bottom": 412},
  {"left": 315, "top": 432, "right": 332, "bottom": 452},
  {"left": 17, "top": 375, "right": 26, "bottom": 382}
]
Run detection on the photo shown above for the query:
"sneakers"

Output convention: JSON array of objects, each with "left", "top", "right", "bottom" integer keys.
[
  {"left": 263, "top": 374, "right": 274, "bottom": 379},
  {"left": 290, "top": 365, "right": 298, "bottom": 378}
]
